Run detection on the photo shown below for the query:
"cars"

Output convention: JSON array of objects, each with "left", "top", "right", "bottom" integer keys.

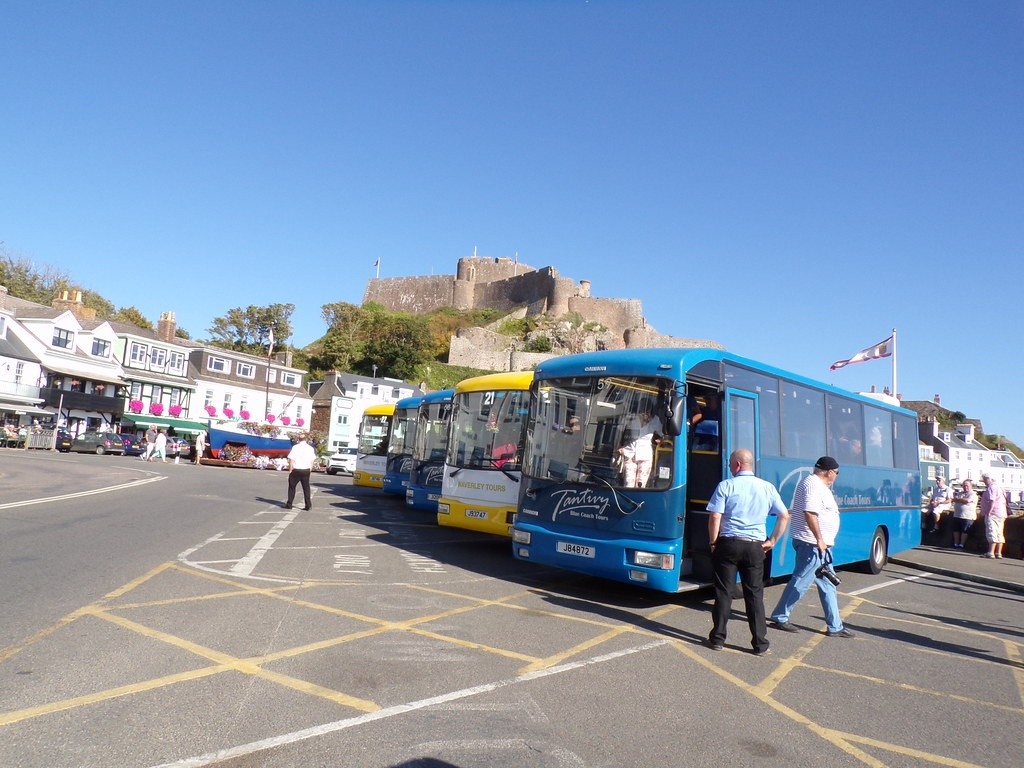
[
  {"left": 165, "top": 437, "right": 190, "bottom": 457},
  {"left": 0, "top": 425, "right": 73, "bottom": 453},
  {"left": 71, "top": 431, "right": 125, "bottom": 455},
  {"left": 109, "top": 433, "right": 146, "bottom": 456},
  {"left": 185, "top": 439, "right": 211, "bottom": 460}
]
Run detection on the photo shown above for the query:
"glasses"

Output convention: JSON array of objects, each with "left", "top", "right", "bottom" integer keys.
[
  {"left": 568, "top": 421, "right": 581, "bottom": 428},
  {"left": 832, "top": 468, "right": 838, "bottom": 475}
]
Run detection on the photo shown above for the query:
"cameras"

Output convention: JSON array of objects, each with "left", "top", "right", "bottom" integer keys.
[{"left": 815, "top": 563, "right": 841, "bottom": 587}]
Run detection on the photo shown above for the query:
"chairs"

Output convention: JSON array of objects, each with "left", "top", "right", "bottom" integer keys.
[{"left": 0, "top": 430, "right": 26, "bottom": 448}]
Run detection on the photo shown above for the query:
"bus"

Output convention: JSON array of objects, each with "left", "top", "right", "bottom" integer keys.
[
  {"left": 351, "top": 404, "right": 396, "bottom": 490},
  {"left": 437, "top": 371, "right": 534, "bottom": 539},
  {"left": 510, "top": 347, "right": 922, "bottom": 599},
  {"left": 382, "top": 397, "right": 422, "bottom": 500},
  {"left": 404, "top": 388, "right": 455, "bottom": 515}
]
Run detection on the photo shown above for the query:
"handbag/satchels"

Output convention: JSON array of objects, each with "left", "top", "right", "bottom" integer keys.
[{"left": 1006, "top": 504, "right": 1013, "bottom": 515}]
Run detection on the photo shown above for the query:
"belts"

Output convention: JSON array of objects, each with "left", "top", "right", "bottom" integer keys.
[{"left": 730, "top": 535, "right": 759, "bottom": 543}]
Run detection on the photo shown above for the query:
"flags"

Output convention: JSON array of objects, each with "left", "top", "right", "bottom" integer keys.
[{"left": 830, "top": 335, "right": 894, "bottom": 371}]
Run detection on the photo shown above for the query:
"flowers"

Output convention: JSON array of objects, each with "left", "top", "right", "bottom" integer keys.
[
  {"left": 168, "top": 404, "right": 182, "bottom": 416},
  {"left": 149, "top": 403, "right": 164, "bottom": 416},
  {"left": 204, "top": 404, "right": 217, "bottom": 416},
  {"left": 240, "top": 410, "right": 250, "bottom": 420},
  {"left": 223, "top": 408, "right": 235, "bottom": 419},
  {"left": 129, "top": 400, "right": 144, "bottom": 412},
  {"left": 267, "top": 414, "right": 276, "bottom": 424},
  {"left": 281, "top": 416, "right": 290, "bottom": 425},
  {"left": 296, "top": 419, "right": 304, "bottom": 426}
]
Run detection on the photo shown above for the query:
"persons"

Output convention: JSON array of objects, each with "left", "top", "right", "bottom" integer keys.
[
  {"left": 922, "top": 475, "right": 952, "bottom": 546},
  {"left": 4, "top": 418, "right": 43, "bottom": 448},
  {"left": 706, "top": 449, "right": 789, "bottom": 657},
  {"left": 700, "top": 390, "right": 722, "bottom": 421},
  {"left": 617, "top": 398, "right": 665, "bottom": 488},
  {"left": 193, "top": 432, "right": 205, "bottom": 466},
  {"left": 951, "top": 479, "right": 978, "bottom": 550},
  {"left": 569, "top": 415, "right": 581, "bottom": 430},
  {"left": 980, "top": 473, "right": 1007, "bottom": 559},
  {"left": 140, "top": 426, "right": 168, "bottom": 463},
  {"left": 285, "top": 434, "right": 317, "bottom": 511},
  {"left": 769, "top": 457, "right": 856, "bottom": 638},
  {"left": 666, "top": 380, "right": 702, "bottom": 425}
]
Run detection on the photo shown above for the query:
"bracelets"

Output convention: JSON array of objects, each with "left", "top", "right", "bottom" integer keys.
[{"left": 709, "top": 542, "right": 716, "bottom": 547}]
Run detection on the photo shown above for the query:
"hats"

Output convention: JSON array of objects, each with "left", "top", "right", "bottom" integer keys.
[
  {"left": 815, "top": 457, "right": 839, "bottom": 470},
  {"left": 935, "top": 476, "right": 945, "bottom": 480}
]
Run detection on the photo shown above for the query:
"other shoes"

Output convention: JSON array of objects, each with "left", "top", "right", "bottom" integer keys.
[
  {"left": 140, "top": 455, "right": 144, "bottom": 461},
  {"left": 995, "top": 553, "right": 1002, "bottom": 558},
  {"left": 163, "top": 461, "right": 167, "bottom": 463},
  {"left": 956, "top": 546, "right": 966, "bottom": 551},
  {"left": 196, "top": 463, "right": 201, "bottom": 465},
  {"left": 708, "top": 639, "right": 723, "bottom": 650},
  {"left": 929, "top": 528, "right": 940, "bottom": 533},
  {"left": 754, "top": 648, "right": 769, "bottom": 655},
  {"left": 952, "top": 545, "right": 958, "bottom": 549},
  {"left": 827, "top": 628, "right": 854, "bottom": 638},
  {"left": 149, "top": 458, "right": 153, "bottom": 462},
  {"left": 983, "top": 552, "right": 995, "bottom": 558},
  {"left": 775, "top": 620, "right": 799, "bottom": 632},
  {"left": 193, "top": 462, "right": 196, "bottom": 465}
]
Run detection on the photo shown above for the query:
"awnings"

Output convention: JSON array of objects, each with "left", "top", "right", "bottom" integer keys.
[
  {"left": 123, "top": 414, "right": 207, "bottom": 436},
  {"left": 0, "top": 398, "right": 55, "bottom": 417},
  {"left": 39, "top": 388, "right": 124, "bottom": 414}
]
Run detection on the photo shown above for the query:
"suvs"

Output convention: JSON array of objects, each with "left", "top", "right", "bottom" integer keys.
[{"left": 326, "top": 447, "right": 359, "bottom": 478}]
